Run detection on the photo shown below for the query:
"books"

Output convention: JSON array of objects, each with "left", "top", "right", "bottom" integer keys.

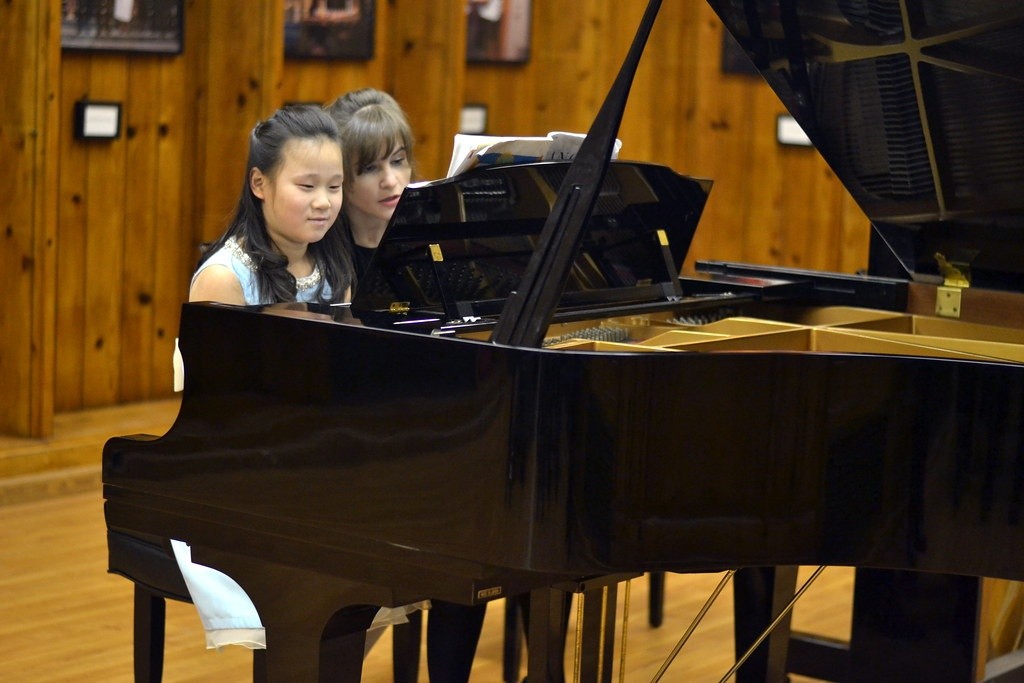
[{"left": 446, "top": 132, "right": 622, "bottom": 178}]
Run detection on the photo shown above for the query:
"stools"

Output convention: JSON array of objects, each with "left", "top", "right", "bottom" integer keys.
[{"left": 106, "top": 527, "right": 423, "bottom": 683}]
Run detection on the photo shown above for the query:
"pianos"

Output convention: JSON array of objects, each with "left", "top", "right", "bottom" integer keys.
[{"left": 101, "top": 0, "right": 1023, "bottom": 683}]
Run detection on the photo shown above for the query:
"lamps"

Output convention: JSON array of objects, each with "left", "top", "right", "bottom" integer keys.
[
  {"left": 774, "top": 110, "right": 816, "bottom": 149},
  {"left": 71, "top": 99, "right": 121, "bottom": 144}
]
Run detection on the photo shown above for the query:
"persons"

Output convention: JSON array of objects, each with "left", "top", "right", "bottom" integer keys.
[{"left": 168, "top": 87, "right": 573, "bottom": 683}]
